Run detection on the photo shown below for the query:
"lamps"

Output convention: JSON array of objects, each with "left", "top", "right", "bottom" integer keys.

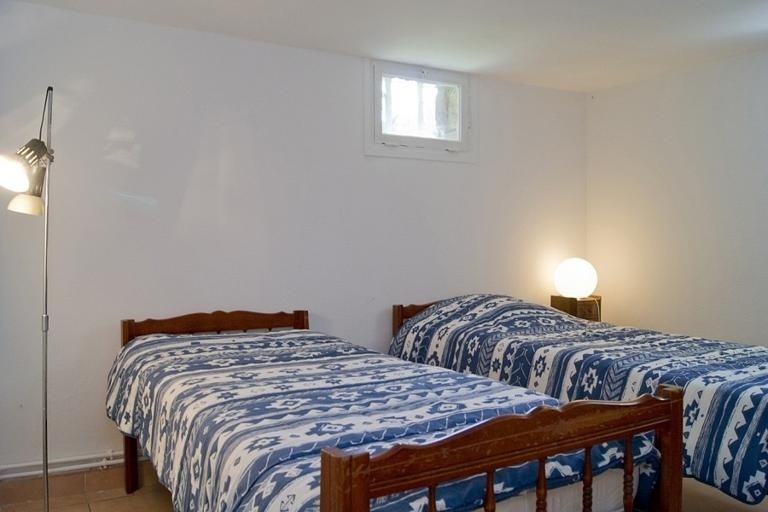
[
  {"left": 552, "top": 258, "right": 599, "bottom": 298},
  {"left": 0, "top": 87, "right": 54, "bottom": 511}
]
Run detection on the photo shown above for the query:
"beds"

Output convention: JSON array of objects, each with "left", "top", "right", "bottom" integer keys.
[
  {"left": 106, "top": 310, "right": 683, "bottom": 511},
  {"left": 386, "top": 293, "right": 768, "bottom": 505}
]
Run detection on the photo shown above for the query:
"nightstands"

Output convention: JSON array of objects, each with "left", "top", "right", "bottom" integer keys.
[{"left": 550, "top": 294, "right": 601, "bottom": 322}]
